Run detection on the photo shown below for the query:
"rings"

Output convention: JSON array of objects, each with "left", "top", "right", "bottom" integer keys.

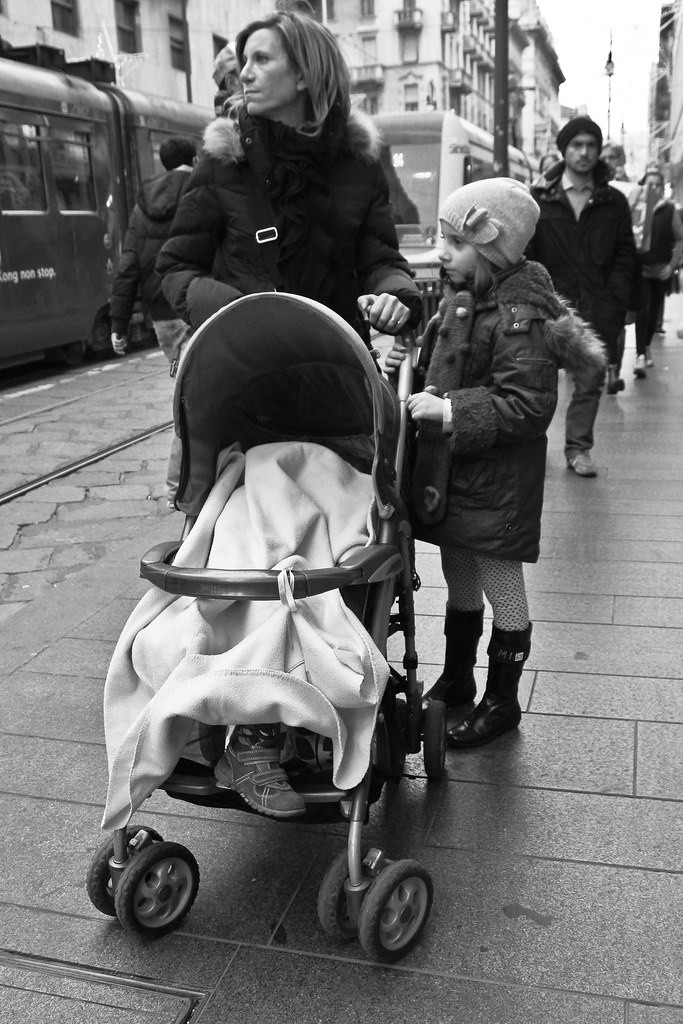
[{"left": 391, "top": 318, "right": 397, "bottom": 322}]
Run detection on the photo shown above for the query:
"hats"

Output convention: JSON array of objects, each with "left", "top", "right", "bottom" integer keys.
[
  {"left": 557, "top": 117, "right": 603, "bottom": 157},
  {"left": 212, "top": 42, "right": 235, "bottom": 87},
  {"left": 440, "top": 177, "right": 541, "bottom": 269}
]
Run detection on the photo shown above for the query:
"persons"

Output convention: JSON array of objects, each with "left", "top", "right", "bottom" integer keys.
[
  {"left": 524, "top": 117, "right": 639, "bottom": 475},
  {"left": 210, "top": 41, "right": 241, "bottom": 95},
  {"left": 383, "top": 178, "right": 608, "bottom": 751},
  {"left": 540, "top": 153, "right": 559, "bottom": 174},
  {"left": 108, "top": 139, "right": 192, "bottom": 513},
  {"left": 602, "top": 146, "right": 683, "bottom": 394},
  {"left": 215, "top": 441, "right": 345, "bottom": 817},
  {"left": 162, "top": 11, "right": 421, "bottom": 370}
]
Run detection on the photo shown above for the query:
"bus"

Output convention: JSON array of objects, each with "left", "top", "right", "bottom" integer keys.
[
  {"left": 371, "top": 109, "right": 546, "bottom": 300},
  {"left": 0, "top": 38, "right": 217, "bottom": 373}
]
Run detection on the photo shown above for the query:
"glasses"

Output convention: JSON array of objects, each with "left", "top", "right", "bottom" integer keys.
[{"left": 600, "top": 156, "right": 619, "bottom": 162}]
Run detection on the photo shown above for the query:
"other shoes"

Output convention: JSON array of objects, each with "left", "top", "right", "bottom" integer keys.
[
  {"left": 654, "top": 324, "right": 665, "bottom": 334},
  {"left": 634, "top": 353, "right": 648, "bottom": 377}
]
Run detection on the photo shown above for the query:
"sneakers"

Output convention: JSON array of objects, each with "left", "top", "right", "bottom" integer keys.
[
  {"left": 568, "top": 452, "right": 596, "bottom": 476},
  {"left": 215, "top": 732, "right": 306, "bottom": 817}
]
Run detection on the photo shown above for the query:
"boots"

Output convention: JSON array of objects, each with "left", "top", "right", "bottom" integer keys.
[
  {"left": 447, "top": 622, "right": 533, "bottom": 747},
  {"left": 607, "top": 365, "right": 625, "bottom": 393},
  {"left": 422, "top": 601, "right": 485, "bottom": 716}
]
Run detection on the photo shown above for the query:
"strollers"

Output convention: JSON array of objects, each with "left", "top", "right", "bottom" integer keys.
[{"left": 85, "top": 303, "right": 450, "bottom": 965}]
